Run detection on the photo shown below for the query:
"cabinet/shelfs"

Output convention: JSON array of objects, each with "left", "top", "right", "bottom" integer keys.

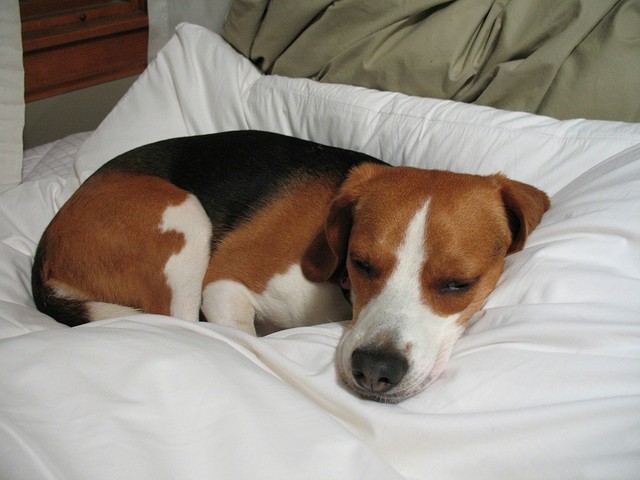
[{"left": 20, "top": 0, "right": 149, "bottom": 102}]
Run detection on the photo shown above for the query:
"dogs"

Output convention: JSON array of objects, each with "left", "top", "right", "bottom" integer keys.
[{"left": 31, "top": 129, "right": 551, "bottom": 404}]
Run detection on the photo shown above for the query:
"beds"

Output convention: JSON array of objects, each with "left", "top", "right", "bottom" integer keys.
[{"left": 0, "top": 0, "right": 640, "bottom": 479}]
